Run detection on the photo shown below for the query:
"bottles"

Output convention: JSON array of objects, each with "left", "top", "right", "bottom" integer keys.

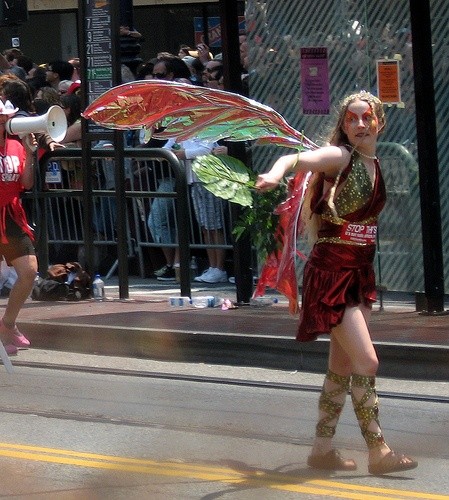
[
  {"left": 33, "top": 272, "right": 41, "bottom": 287},
  {"left": 189, "top": 256, "right": 199, "bottom": 282},
  {"left": 193, "top": 296, "right": 225, "bottom": 309},
  {"left": 250, "top": 297, "right": 278, "bottom": 307},
  {"left": 92, "top": 274, "right": 106, "bottom": 301},
  {"left": 169, "top": 297, "right": 193, "bottom": 306}
]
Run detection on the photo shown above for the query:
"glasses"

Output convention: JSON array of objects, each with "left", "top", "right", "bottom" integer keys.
[
  {"left": 46, "top": 68, "right": 53, "bottom": 72},
  {"left": 58, "top": 90, "right": 67, "bottom": 94},
  {"left": 218, "top": 82, "right": 223, "bottom": 85},
  {"left": 151, "top": 72, "right": 170, "bottom": 79},
  {"left": 203, "top": 67, "right": 213, "bottom": 73},
  {"left": 208, "top": 76, "right": 215, "bottom": 81}
]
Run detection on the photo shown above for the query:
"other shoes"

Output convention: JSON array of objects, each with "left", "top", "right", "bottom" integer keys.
[
  {"left": 0, "top": 318, "right": 30, "bottom": 346},
  {"left": 3, "top": 344, "right": 18, "bottom": 354},
  {"left": 153, "top": 265, "right": 170, "bottom": 277},
  {"left": 191, "top": 256, "right": 197, "bottom": 268}
]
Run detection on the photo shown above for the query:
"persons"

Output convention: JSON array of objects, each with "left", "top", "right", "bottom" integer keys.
[
  {"left": 0, "top": 26, "right": 411, "bottom": 356},
  {"left": 251, "top": 90, "right": 418, "bottom": 475}
]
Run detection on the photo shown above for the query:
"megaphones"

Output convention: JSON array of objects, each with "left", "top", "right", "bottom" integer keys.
[{"left": 4, "top": 105, "right": 68, "bottom": 143}]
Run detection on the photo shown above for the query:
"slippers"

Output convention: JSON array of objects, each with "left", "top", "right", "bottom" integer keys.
[
  {"left": 308, "top": 449, "right": 356, "bottom": 471},
  {"left": 368, "top": 450, "right": 417, "bottom": 474}
]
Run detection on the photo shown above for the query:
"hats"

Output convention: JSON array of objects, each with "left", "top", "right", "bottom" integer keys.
[{"left": 0, "top": 100, "right": 19, "bottom": 115}]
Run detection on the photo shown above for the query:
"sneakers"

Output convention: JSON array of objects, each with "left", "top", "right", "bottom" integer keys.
[
  {"left": 157, "top": 269, "right": 176, "bottom": 280},
  {"left": 194, "top": 266, "right": 228, "bottom": 283}
]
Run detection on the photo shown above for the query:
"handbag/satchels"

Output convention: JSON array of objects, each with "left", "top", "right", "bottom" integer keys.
[{"left": 0, "top": 255, "right": 92, "bottom": 302}]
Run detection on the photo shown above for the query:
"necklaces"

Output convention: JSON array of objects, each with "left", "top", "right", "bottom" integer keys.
[{"left": 346, "top": 144, "right": 377, "bottom": 160}]
